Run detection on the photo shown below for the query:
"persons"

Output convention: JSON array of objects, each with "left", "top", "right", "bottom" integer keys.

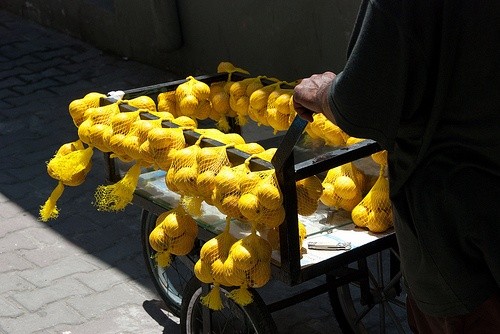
[{"left": 292, "top": 0, "right": 500, "bottom": 334}]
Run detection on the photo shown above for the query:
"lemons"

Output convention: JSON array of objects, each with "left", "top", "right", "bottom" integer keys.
[{"left": 46, "top": 61, "right": 395, "bottom": 287}]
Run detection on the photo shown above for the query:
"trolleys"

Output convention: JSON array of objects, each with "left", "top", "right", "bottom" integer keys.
[{"left": 99, "top": 70, "right": 412, "bottom": 334}]
